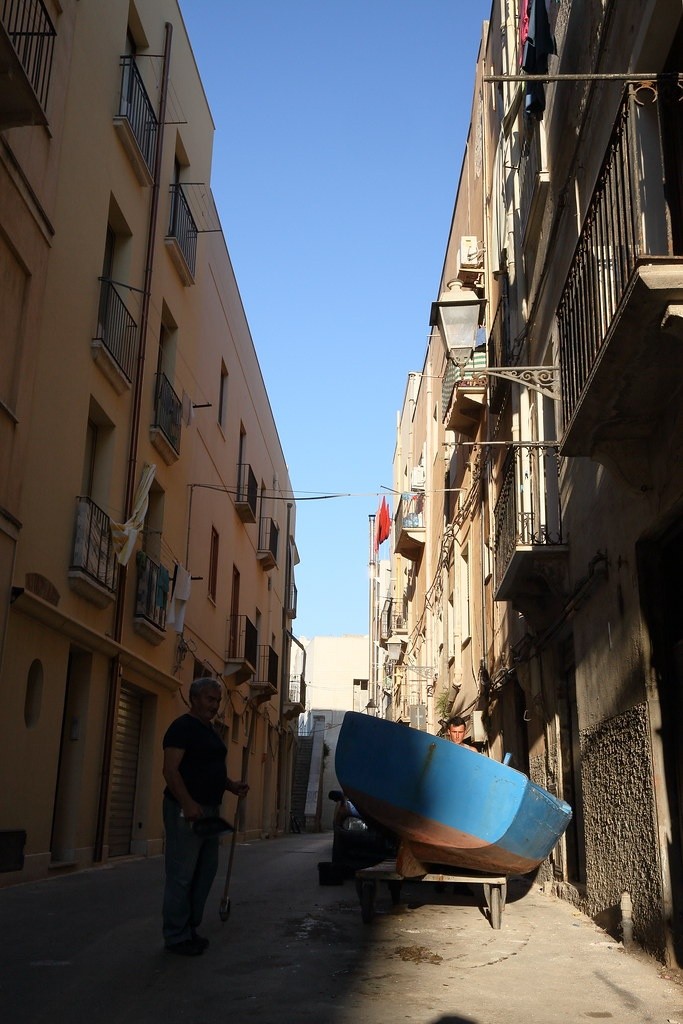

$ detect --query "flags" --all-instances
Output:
[378,496,390,544]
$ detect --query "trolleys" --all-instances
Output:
[356,858,511,930]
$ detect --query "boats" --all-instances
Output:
[335,711,575,878]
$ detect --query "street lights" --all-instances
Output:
[365,698,380,717]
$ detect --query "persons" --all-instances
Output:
[159,678,250,958]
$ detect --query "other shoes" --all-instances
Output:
[165,932,211,958]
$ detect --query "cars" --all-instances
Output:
[329,790,399,875]
